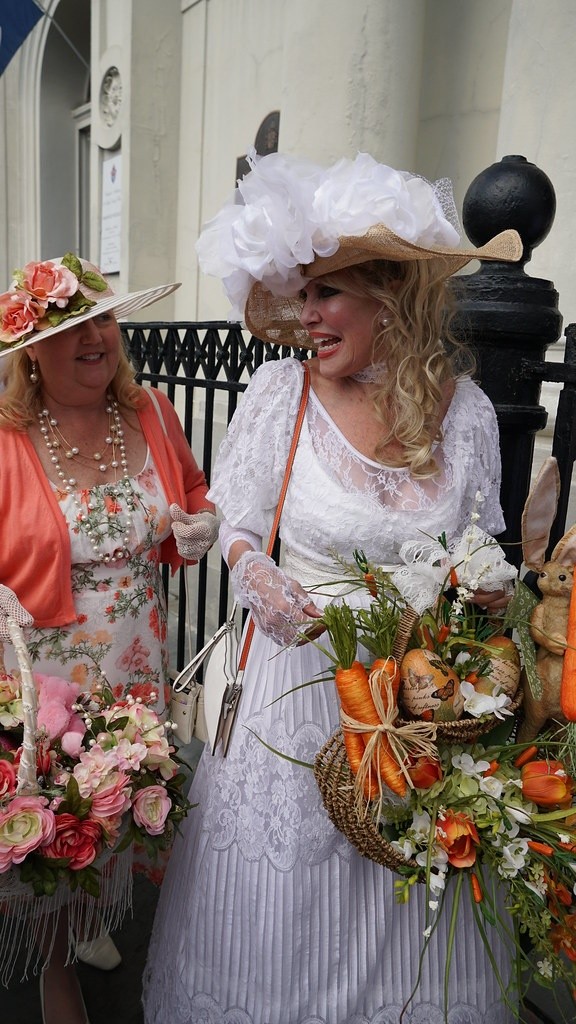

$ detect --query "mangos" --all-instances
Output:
[471,635,520,698]
[401,649,467,720]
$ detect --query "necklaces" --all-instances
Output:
[33,391,133,563]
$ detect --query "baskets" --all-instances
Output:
[314,601,523,879]
[0,614,132,920]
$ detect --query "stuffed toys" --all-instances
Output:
[513,457,575,745]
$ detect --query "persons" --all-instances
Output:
[140,150,528,1024]
[0,250,221,1024]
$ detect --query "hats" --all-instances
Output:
[0,252,182,357]
[193,154,522,350]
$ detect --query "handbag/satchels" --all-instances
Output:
[172,625,244,758]
[167,669,201,745]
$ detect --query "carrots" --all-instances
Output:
[333,657,406,802]
